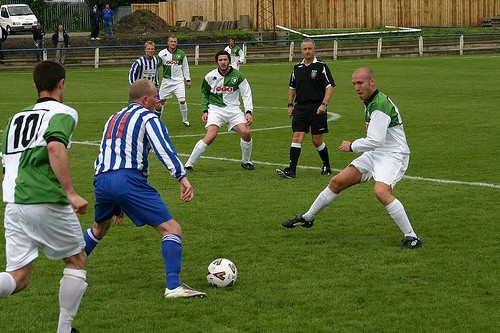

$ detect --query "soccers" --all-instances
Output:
[206,258,237,288]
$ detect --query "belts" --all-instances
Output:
[58,41,64,42]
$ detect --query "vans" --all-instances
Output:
[0,3,41,35]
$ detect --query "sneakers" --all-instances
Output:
[282,214,315,228]
[401,235,422,250]
[164,282,207,300]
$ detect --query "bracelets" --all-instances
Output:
[321,102,327,106]
[288,103,294,108]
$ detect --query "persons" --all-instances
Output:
[281,67,422,248]
[52,24,69,65]
[0,61,89,333]
[225,39,245,70]
[80,77,206,299]
[33,23,45,61]
[157,36,191,128]
[88,4,101,41]
[186,51,254,171]
[274,38,336,177]
[102,6,114,39]
[128,41,162,119]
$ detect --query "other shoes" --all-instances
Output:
[321,165,332,175]
[91,37,95,40]
[276,167,296,178]
[183,119,190,127]
[240,161,256,170]
[96,37,101,40]
[184,166,193,171]
